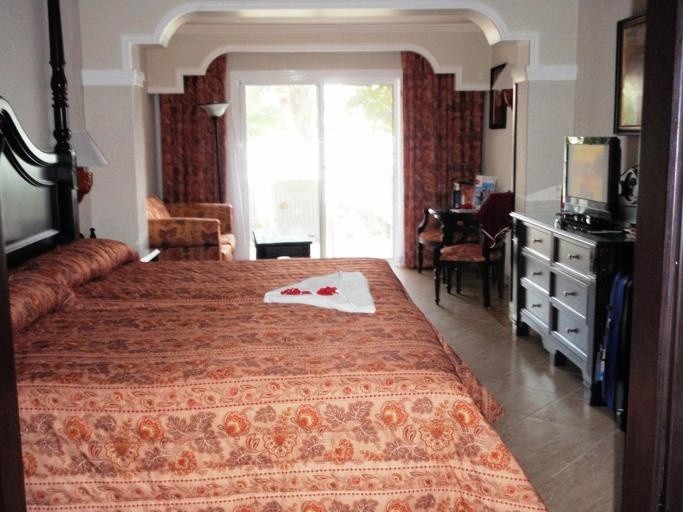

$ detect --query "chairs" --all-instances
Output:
[416,181,514,310]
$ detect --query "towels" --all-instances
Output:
[261,271,377,314]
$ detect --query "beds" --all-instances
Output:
[0,0,549,512]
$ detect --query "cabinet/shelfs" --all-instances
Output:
[509,208,636,406]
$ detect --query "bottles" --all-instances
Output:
[453,182,460,208]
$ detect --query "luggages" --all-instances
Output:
[602,272,634,433]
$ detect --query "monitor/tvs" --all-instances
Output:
[560,136,622,229]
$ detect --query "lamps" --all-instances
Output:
[200,99,230,203]
[75,127,109,203]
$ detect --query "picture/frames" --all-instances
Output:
[489,62,507,130]
[613,13,648,135]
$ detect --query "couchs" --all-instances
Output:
[146,194,236,261]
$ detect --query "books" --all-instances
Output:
[472,174,496,211]
